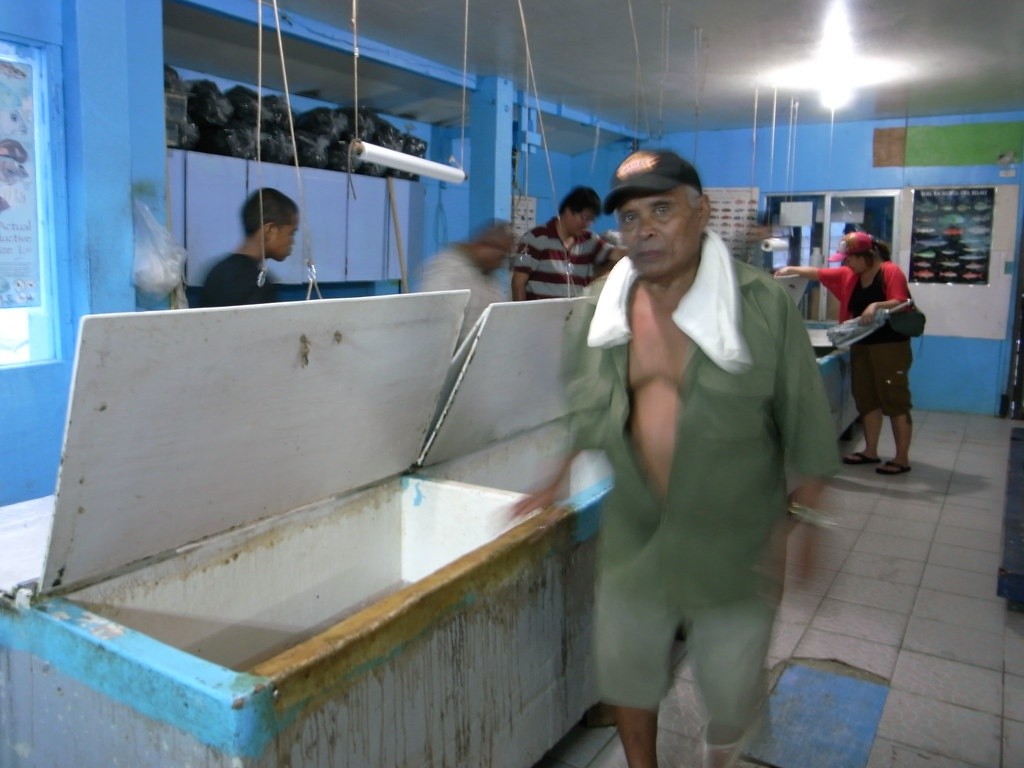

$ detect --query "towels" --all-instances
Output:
[586,224,752,374]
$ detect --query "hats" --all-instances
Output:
[602,150,702,217]
[828,232,873,262]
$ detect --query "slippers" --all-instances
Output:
[843,452,882,464]
[875,460,911,474]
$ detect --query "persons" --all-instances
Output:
[774,231,926,475]
[841,224,858,265]
[412,219,514,350]
[509,148,844,768]
[200,187,299,308]
[510,186,625,300]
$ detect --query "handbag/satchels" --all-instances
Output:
[890,311,927,338]
